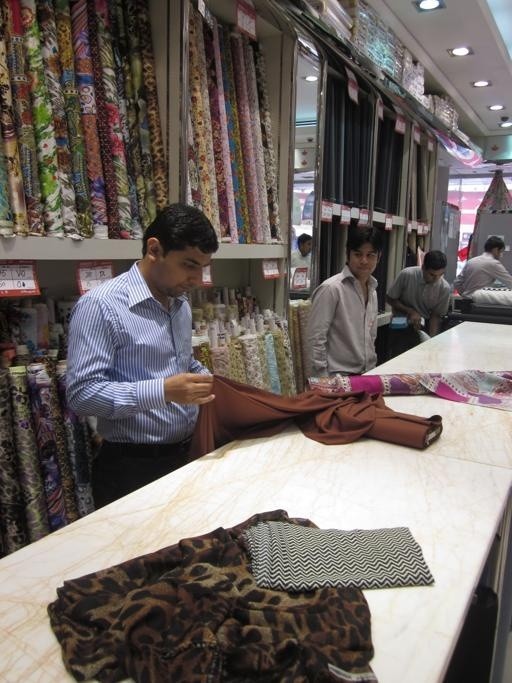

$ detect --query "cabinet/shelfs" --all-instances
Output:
[0,1,291,560]
[321,36,438,364]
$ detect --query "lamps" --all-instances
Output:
[499,116,511,128]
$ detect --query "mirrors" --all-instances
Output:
[289,34,323,298]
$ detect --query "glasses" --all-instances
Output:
[499,116,511,128]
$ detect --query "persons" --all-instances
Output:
[64,201,222,511]
[453,235,512,313]
[291,233,313,269]
[384,250,451,358]
[304,224,384,380]
